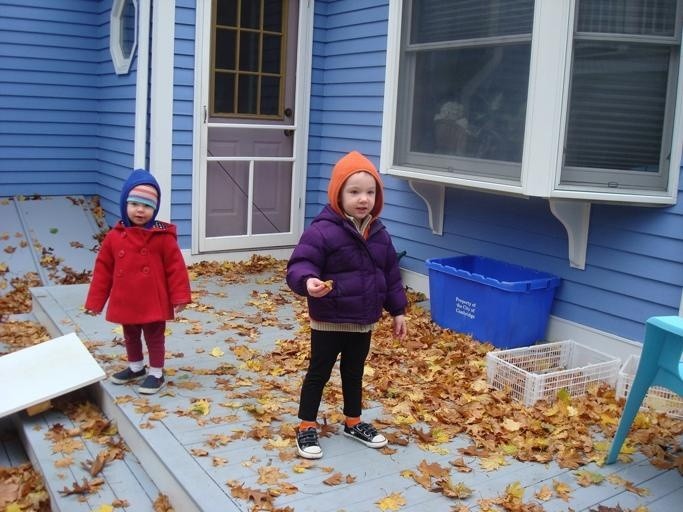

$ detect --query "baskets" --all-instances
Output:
[487,340,621,407]
[615,354,683,419]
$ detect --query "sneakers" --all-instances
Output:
[137,374,165,394]
[343,421,388,448]
[111,366,146,385]
[296,427,322,458]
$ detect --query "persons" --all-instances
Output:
[81,168,192,396]
[284,150,409,460]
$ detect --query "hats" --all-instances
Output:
[127,184,158,210]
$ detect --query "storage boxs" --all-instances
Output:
[425,252,564,353]
